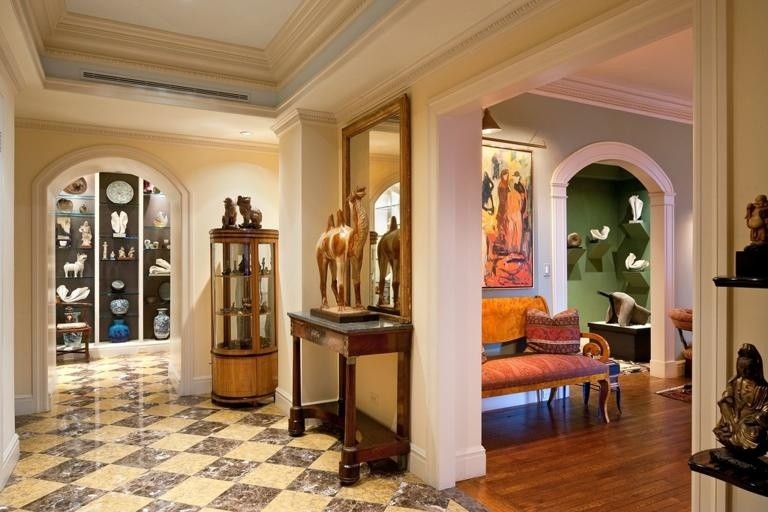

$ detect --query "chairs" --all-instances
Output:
[54,301,93,364]
[665,308,692,390]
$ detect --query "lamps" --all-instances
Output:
[479,109,505,133]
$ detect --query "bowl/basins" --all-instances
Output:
[112,279,125,289]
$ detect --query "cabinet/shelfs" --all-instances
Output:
[99,173,139,343]
[687,277,768,497]
[144,180,171,339]
[208,228,280,408]
[620,222,650,288]
[55,195,96,344]
[566,236,612,263]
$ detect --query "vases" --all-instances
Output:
[153,308,171,340]
[109,297,130,315]
[107,319,132,342]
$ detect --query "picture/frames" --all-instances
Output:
[482,140,536,290]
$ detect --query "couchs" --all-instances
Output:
[482,296,610,425]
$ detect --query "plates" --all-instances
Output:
[63,177,88,195]
[158,281,170,302]
[105,180,136,204]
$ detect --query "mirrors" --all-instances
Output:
[339,95,412,324]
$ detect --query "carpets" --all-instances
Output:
[656,383,692,404]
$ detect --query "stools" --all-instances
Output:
[582,356,624,418]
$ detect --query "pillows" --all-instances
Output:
[524,306,581,355]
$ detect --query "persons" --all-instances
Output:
[496,169,527,256]
[713,345,767,459]
[745,193,767,244]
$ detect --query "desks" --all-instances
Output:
[288,312,409,484]
[586,319,650,362]
